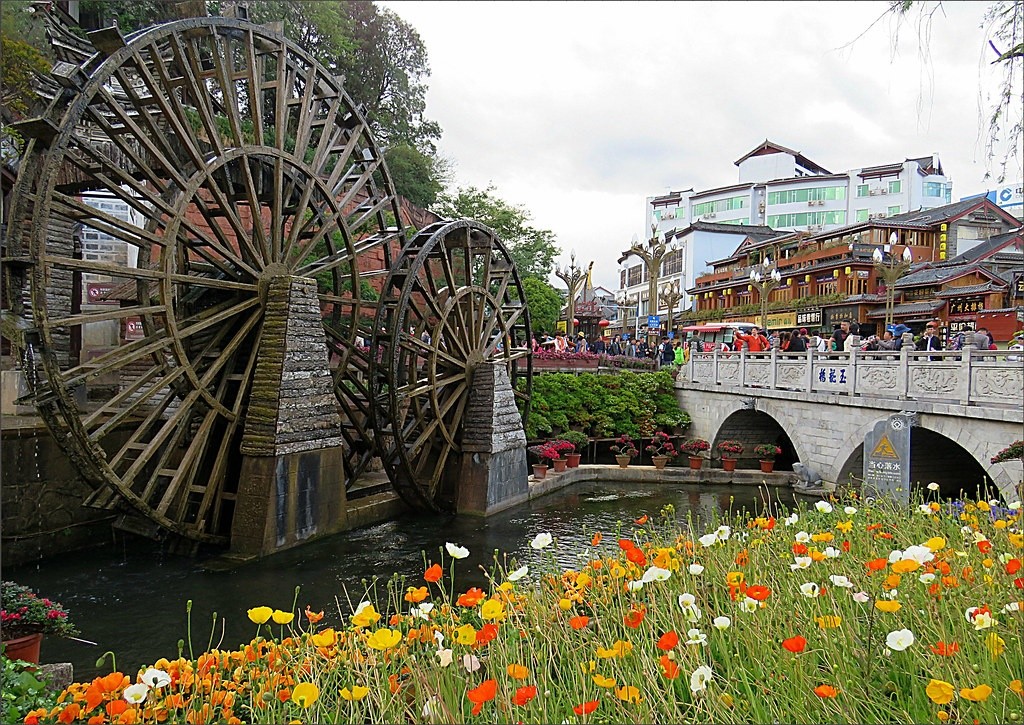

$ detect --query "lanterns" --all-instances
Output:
[748,285,752,292]
[941,224,947,231]
[573,318,579,327]
[709,292,713,298]
[939,252,946,259]
[598,319,610,328]
[690,296,693,301]
[722,289,726,296]
[940,243,946,251]
[833,270,838,278]
[695,295,698,300]
[727,289,731,294]
[845,267,851,274]
[786,278,791,286]
[704,293,708,299]
[805,275,810,283]
[939,233,946,242]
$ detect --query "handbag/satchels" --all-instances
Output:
[760,342,765,350]
[664,351,675,361]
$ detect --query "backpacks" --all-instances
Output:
[949,333,963,349]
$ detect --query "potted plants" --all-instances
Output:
[555,431,590,467]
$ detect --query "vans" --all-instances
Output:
[705,322,762,333]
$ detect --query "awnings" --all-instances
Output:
[682,325,726,332]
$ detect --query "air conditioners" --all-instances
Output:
[808,225,823,229]
[807,200,824,205]
[760,202,765,213]
[868,213,886,218]
[661,214,674,220]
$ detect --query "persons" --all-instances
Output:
[341,324,432,346]
[1009,334,1024,350]
[730,319,997,362]
[687,330,705,352]
[516,330,691,374]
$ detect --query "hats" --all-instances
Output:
[800,328,807,334]
[894,324,911,336]
[1018,334,1024,339]
[578,332,584,337]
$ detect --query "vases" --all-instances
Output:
[532,465,549,479]
[721,459,737,471]
[652,457,668,469]
[553,459,568,472]
[759,460,775,472]
[615,455,630,468]
[688,456,704,469]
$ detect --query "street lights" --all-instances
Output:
[657,276,683,343]
[614,290,638,349]
[873,232,912,333]
[555,248,594,342]
[631,214,678,348]
[750,257,781,330]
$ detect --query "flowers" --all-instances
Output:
[715,439,745,457]
[753,444,781,459]
[528,445,559,464]
[609,434,639,456]
[646,431,676,455]
[681,439,711,455]
[543,440,575,459]
